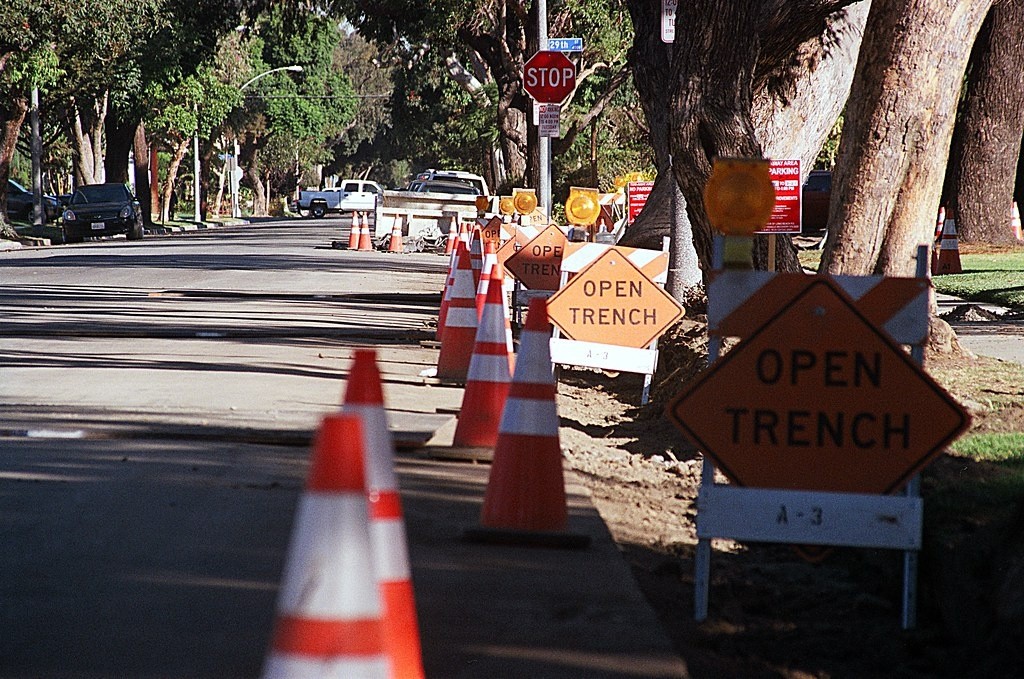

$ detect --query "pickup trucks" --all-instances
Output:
[293,179,385,219]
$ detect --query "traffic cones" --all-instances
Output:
[255,412,403,679]
[418,263,510,465]
[451,297,597,555]
[344,210,358,250]
[330,347,429,679]
[421,220,518,387]
[935,206,948,243]
[357,212,377,252]
[436,215,458,256]
[383,212,409,254]
[1012,201,1022,239]
[934,208,964,276]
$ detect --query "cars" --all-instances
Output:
[61,183,144,243]
[6,179,61,225]
[393,169,489,196]
[800,171,835,230]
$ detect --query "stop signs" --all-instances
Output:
[522,50,577,106]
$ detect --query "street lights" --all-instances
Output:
[228,66,303,216]
